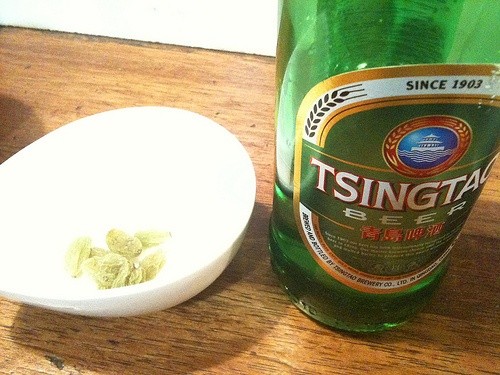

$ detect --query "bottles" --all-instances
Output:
[267,0,500,331]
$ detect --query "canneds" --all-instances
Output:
[271,0,500,333]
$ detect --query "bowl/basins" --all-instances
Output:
[0,106,256,317]
